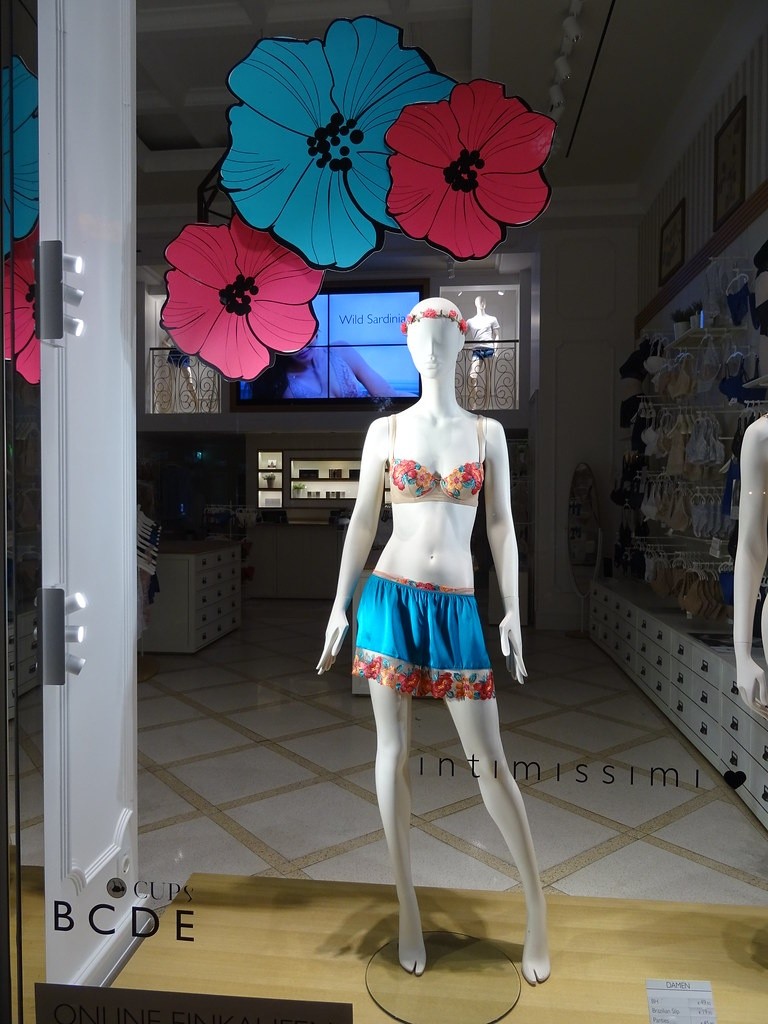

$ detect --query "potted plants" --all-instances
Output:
[263,474,276,489]
[293,482,306,498]
[686,301,702,328]
[670,308,688,340]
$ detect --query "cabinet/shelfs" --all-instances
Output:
[137,540,243,653]
[14,595,41,698]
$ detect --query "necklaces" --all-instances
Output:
[287,356,323,396]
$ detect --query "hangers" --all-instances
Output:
[613,396,767,602]
[637,254,759,368]
[381,503,392,519]
[234,505,260,515]
[205,504,233,514]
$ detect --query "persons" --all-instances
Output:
[241,297,419,399]
[314,294,557,988]
[733,411,768,717]
[463,294,501,412]
[161,334,202,414]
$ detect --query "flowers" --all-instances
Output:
[400,307,468,334]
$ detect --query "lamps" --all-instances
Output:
[64,652,85,675]
[64,593,86,615]
[65,626,84,643]
